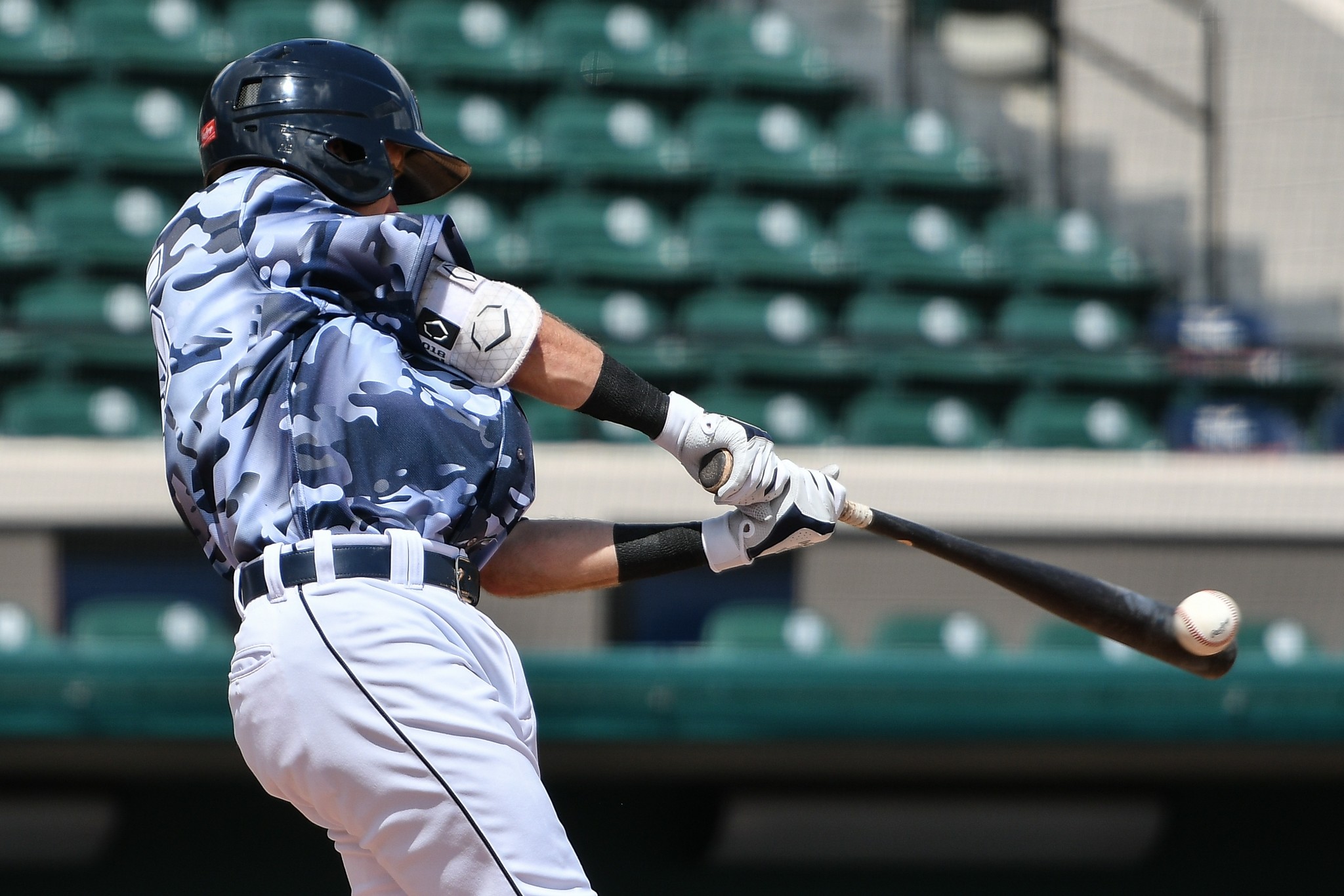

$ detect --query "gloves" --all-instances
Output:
[651,390,791,506]
[702,464,847,572]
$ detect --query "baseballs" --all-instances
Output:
[1174,589,1240,657]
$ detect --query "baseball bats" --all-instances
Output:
[698,443,1240,680]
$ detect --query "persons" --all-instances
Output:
[145,39,847,896]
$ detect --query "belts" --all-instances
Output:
[237,545,480,606]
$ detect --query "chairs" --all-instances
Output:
[0,0,1344,462]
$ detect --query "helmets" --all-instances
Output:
[198,36,473,208]
